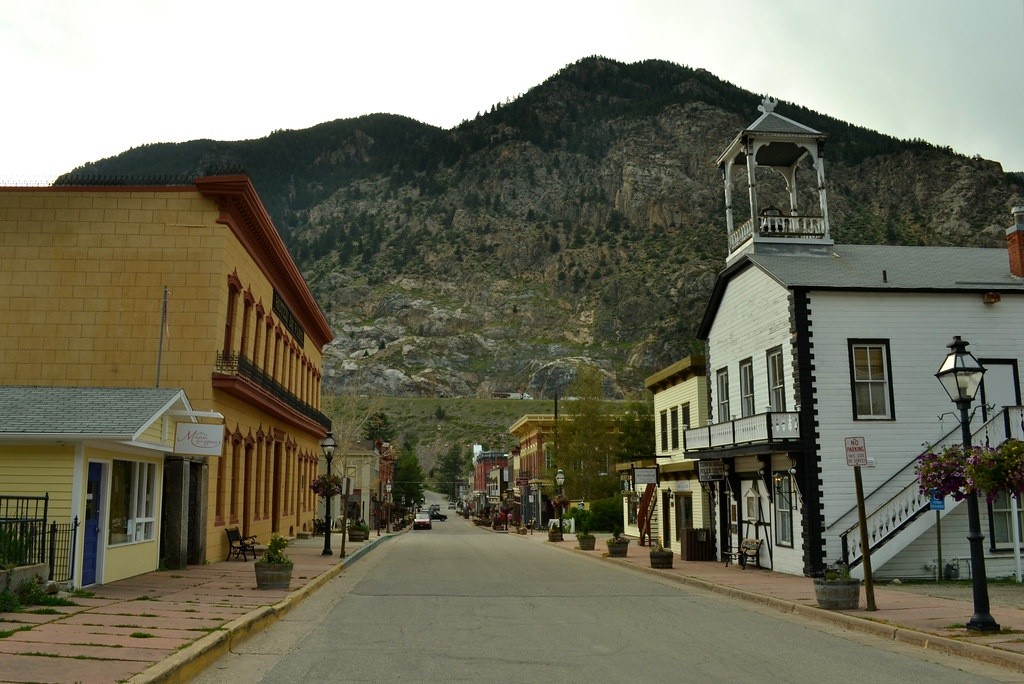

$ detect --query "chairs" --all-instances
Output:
[813,564,860,610]
[312,518,325,537]
[724,538,763,570]
[224,526,256,562]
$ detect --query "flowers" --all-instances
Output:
[650,536,669,552]
[607,523,630,543]
[914,438,1024,503]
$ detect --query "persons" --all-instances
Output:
[349,499,362,521]
[499,512,506,525]
[507,511,513,526]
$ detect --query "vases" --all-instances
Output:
[650,551,673,569]
[607,540,628,557]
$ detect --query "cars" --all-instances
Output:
[421,510,447,521]
[413,513,432,529]
[448,503,456,509]
[430,504,440,511]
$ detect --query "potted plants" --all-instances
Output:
[348,519,369,541]
[548,522,561,542]
[576,527,596,550]
[255,535,293,590]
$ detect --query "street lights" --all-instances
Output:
[933,335,1001,630]
[385,479,391,533]
[320,432,338,556]
[502,491,508,530]
[401,496,405,526]
[555,470,565,540]
[411,498,414,514]
